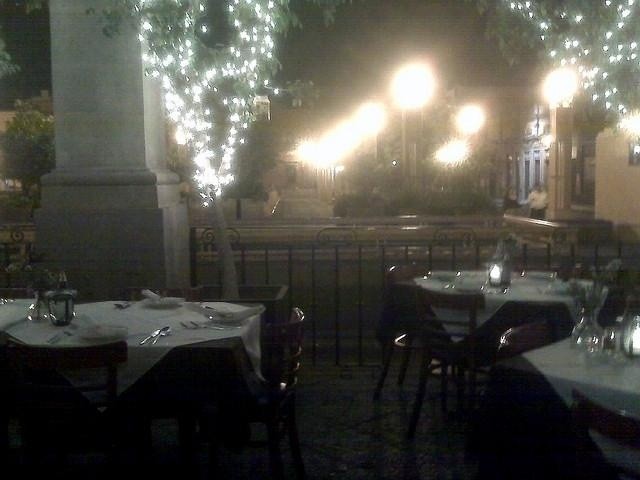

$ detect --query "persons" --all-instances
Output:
[492,186,524,260]
[518,180,551,219]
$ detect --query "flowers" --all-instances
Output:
[4,262,70,295]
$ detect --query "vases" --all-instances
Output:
[26,293,51,324]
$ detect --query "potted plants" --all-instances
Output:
[83,0,303,323]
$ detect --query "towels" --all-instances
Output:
[140,288,253,319]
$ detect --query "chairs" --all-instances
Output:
[0,337,134,480]
[193,306,310,479]
[367,254,640,480]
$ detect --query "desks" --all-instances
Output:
[1,293,267,480]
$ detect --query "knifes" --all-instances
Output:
[139,328,160,345]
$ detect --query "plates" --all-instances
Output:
[204,305,266,324]
[78,325,129,341]
[142,296,186,309]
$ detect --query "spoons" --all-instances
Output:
[151,326,171,345]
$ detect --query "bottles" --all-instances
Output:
[25,290,49,322]
[568,305,604,353]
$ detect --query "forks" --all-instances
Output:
[177,319,241,331]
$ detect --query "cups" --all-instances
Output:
[605,323,630,366]
[542,269,558,283]
[581,331,601,364]
